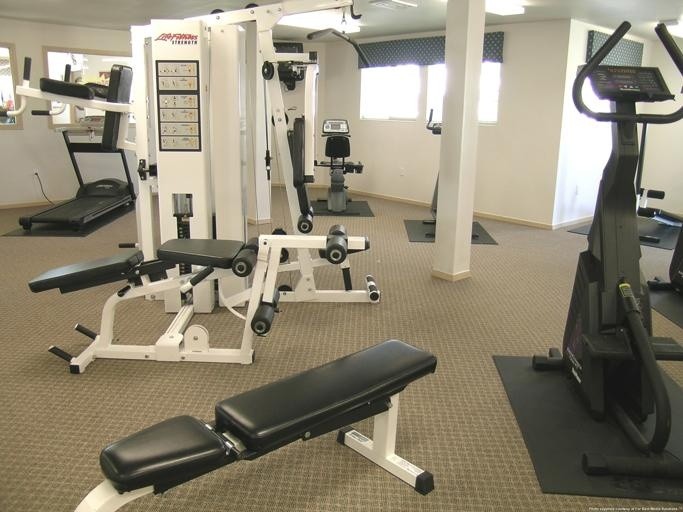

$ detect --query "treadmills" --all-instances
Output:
[18,116,137,237]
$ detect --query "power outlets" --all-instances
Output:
[34,170,39,177]
